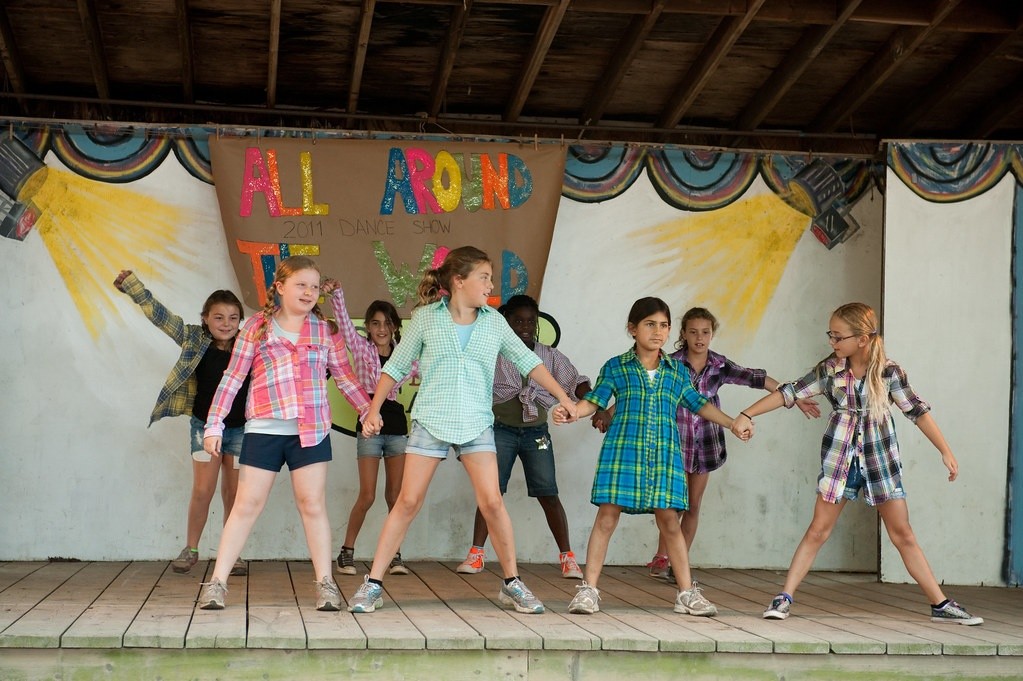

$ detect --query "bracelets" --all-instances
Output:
[740,412,751,420]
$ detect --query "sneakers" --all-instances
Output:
[172,545,200,575]
[229,555,250,576]
[559,550,584,579]
[313,576,341,611]
[389,553,409,574]
[498,574,546,613]
[197,576,229,609]
[667,565,677,583]
[762,591,794,619]
[568,579,602,613]
[348,573,384,613]
[673,581,719,616]
[930,598,984,625]
[336,545,357,575]
[456,547,487,573]
[647,553,670,579]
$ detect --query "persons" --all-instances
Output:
[112,268,254,576]
[347,244,580,614]
[322,274,409,576]
[456,298,609,579]
[729,302,984,624]
[590,306,821,584]
[552,295,748,617]
[200,254,384,611]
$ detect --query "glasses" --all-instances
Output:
[826,330,861,343]
